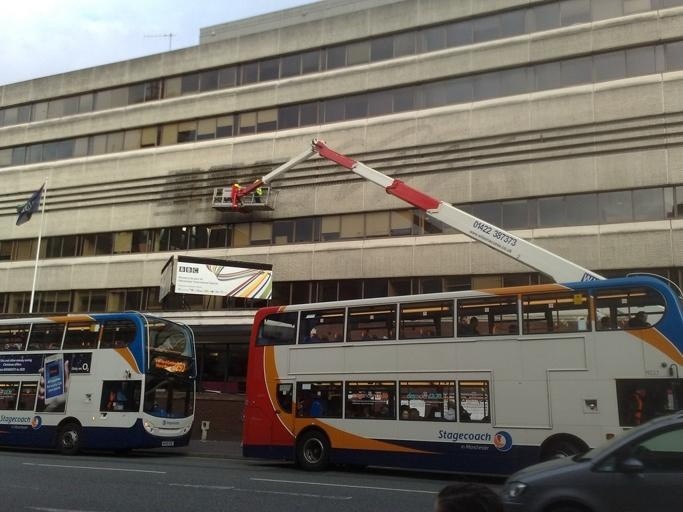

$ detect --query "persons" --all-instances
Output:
[283,387,420,420]
[626,310,653,327]
[459,316,473,336]
[232,179,244,205]
[254,177,263,204]
[117,390,130,401]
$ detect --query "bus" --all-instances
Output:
[0,310,197,456]
[243,275,683,470]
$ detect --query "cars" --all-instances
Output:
[501,410,683,512]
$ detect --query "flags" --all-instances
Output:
[16,181,45,226]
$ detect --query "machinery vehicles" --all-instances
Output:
[210,139,609,283]
